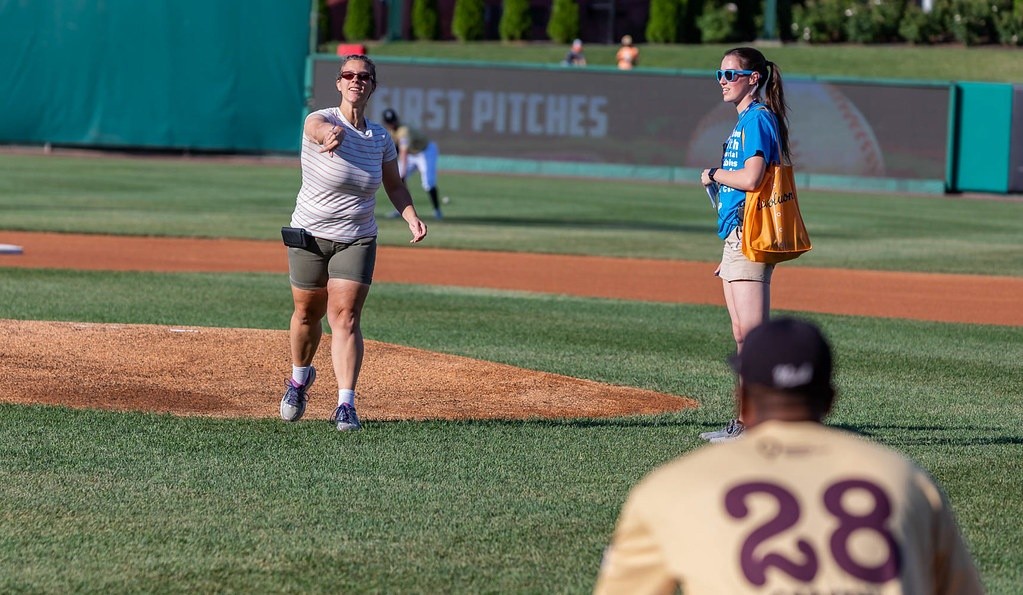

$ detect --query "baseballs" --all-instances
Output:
[442,197,450,205]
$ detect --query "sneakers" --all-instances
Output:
[329,403,360,430]
[280,365,316,422]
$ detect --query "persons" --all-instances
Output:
[565,39,586,66]
[380,108,444,218]
[698,45,788,442]
[593,317,986,595]
[281,54,426,430]
[615,35,639,69]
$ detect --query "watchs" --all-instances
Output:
[708,167,718,182]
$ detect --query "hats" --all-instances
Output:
[728,316,833,390]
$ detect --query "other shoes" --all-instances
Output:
[700,419,745,444]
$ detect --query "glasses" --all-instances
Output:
[338,71,371,81]
[716,70,754,82]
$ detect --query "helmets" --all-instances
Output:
[384,109,396,122]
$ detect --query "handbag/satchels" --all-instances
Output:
[741,106,812,262]
